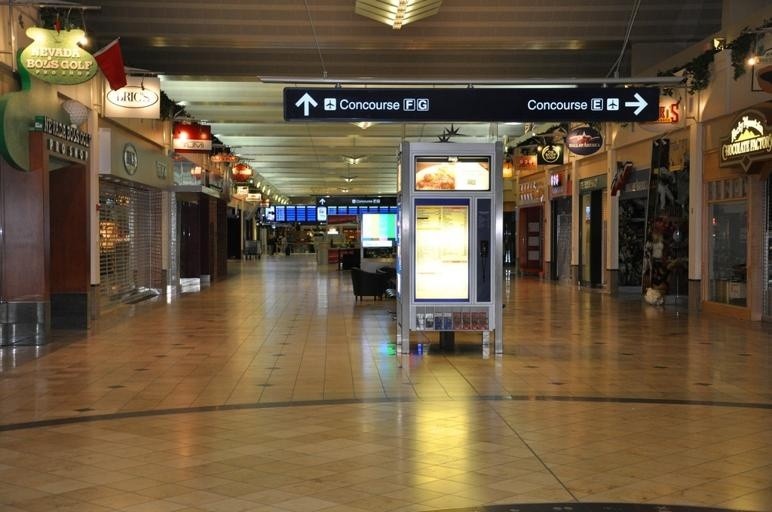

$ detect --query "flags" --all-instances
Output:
[93,40,128,91]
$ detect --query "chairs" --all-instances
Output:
[376,268,395,298]
[350,267,387,300]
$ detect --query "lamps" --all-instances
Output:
[337,137,368,193]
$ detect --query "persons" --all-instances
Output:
[644,281,665,306]
[267,233,324,256]
